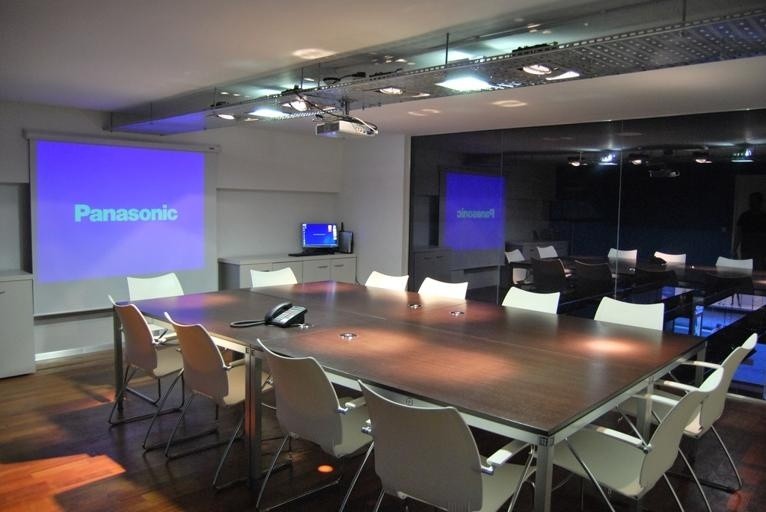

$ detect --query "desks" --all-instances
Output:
[115,281,710,512]
[0,269,37,380]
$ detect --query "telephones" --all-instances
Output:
[265,301,307,327]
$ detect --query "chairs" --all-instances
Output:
[364,270,410,292]
[108,294,229,452]
[611,333,757,512]
[529,367,724,512]
[501,245,754,305]
[501,286,561,313]
[356,377,537,512]
[163,310,294,490]
[249,266,299,288]
[254,338,375,511]
[124,273,185,404]
[417,277,469,300]
[594,296,665,332]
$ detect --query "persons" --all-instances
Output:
[732,192,765,273]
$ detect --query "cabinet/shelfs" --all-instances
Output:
[303,257,356,283]
[241,261,302,288]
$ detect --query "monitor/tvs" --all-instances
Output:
[302,223,339,254]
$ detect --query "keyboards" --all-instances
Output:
[289,252,328,257]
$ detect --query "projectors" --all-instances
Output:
[314,117,379,138]
